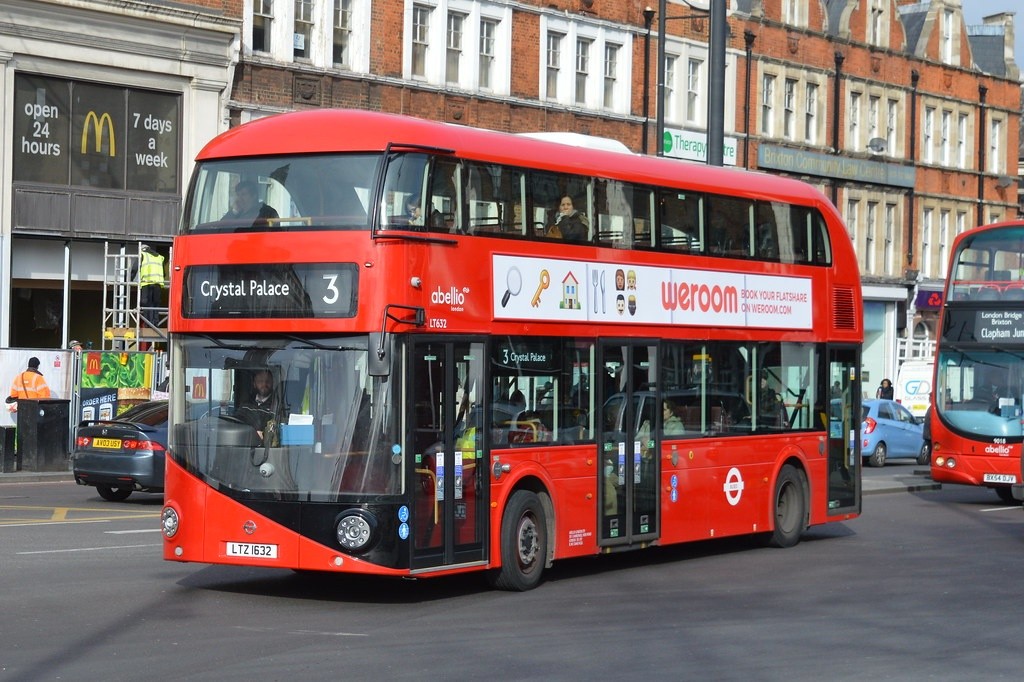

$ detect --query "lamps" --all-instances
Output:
[994,175,1012,189]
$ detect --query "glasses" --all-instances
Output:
[408,207,417,214]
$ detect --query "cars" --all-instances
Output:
[861,398,931,466]
[450,398,750,447]
[72,399,274,500]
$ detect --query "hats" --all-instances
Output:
[67,340,82,349]
[141,244,151,249]
[28,357,40,367]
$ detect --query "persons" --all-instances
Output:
[219,370,277,440]
[220,181,280,228]
[11,357,50,399]
[634,399,685,452]
[543,192,589,242]
[404,192,448,227]
[876,378,894,400]
[130,244,167,327]
[830,380,842,399]
[761,372,789,427]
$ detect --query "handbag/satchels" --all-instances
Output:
[547,227,563,240]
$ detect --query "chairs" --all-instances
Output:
[488,401,792,440]
[975,284,1024,300]
[387,212,757,260]
[277,380,305,416]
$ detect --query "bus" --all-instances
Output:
[931,220,1024,506]
[159,107,863,593]
